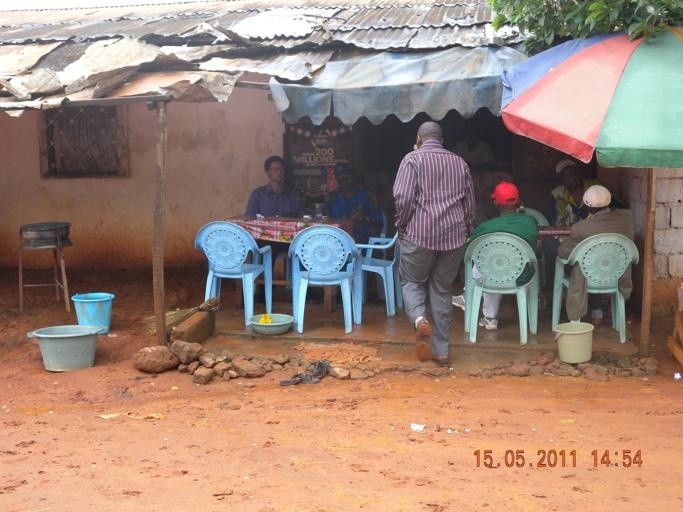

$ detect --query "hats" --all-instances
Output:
[489,181,520,207]
[555,158,576,175]
[582,184,612,208]
[334,163,352,181]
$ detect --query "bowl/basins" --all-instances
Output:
[16,221,78,244]
[247,313,293,335]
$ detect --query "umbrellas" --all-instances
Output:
[498,17,683,357]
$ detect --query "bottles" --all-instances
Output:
[518,202,525,214]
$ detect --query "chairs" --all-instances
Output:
[550,232,639,344]
[194,221,273,326]
[288,225,363,334]
[378,209,389,299]
[463,232,540,346]
[355,230,403,317]
[274,250,295,290]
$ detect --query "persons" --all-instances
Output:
[324,161,382,303]
[243,156,304,304]
[542,184,636,326]
[548,159,625,228]
[392,122,477,367]
[451,181,540,331]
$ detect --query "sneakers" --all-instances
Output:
[478,316,498,330]
[431,353,451,367]
[415,320,432,362]
[452,295,466,311]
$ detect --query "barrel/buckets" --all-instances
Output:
[71,292,116,335]
[552,318,595,364]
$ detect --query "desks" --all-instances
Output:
[225,215,367,313]
[469,223,572,252]
